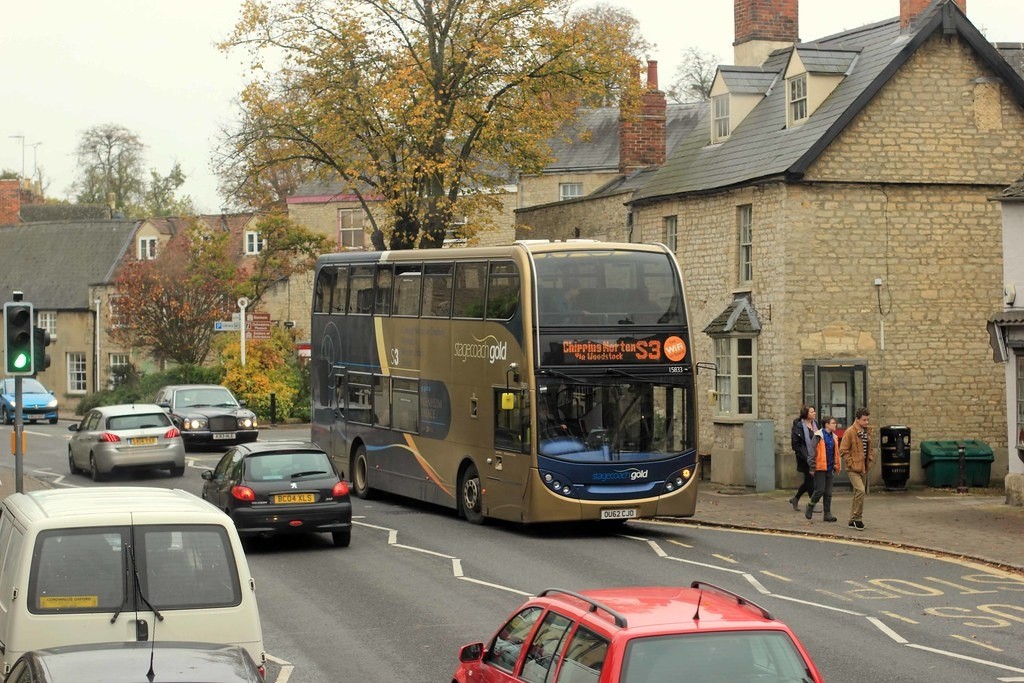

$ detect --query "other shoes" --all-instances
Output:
[825,513,837,521]
[810,507,822,513]
[806,503,813,519]
[849,520,867,531]
[790,497,800,510]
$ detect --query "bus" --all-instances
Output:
[309,237,719,538]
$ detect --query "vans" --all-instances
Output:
[0,486,268,683]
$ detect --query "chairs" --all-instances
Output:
[144,533,173,549]
[353,285,662,321]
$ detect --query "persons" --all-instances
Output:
[804,415,841,522]
[547,287,590,324]
[839,406,874,530]
[537,401,568,438]
[788,404,822,512]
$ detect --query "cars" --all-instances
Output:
[151,385,258,453]
[200,440,354,551]
[449,579,825,683]
[0,378,58,425]
[68,404,186,483]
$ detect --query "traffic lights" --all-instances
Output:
[4,302,34,376]
[33,325,52,373]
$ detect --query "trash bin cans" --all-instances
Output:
[879,424,912,491]
[918,440,995,487]
